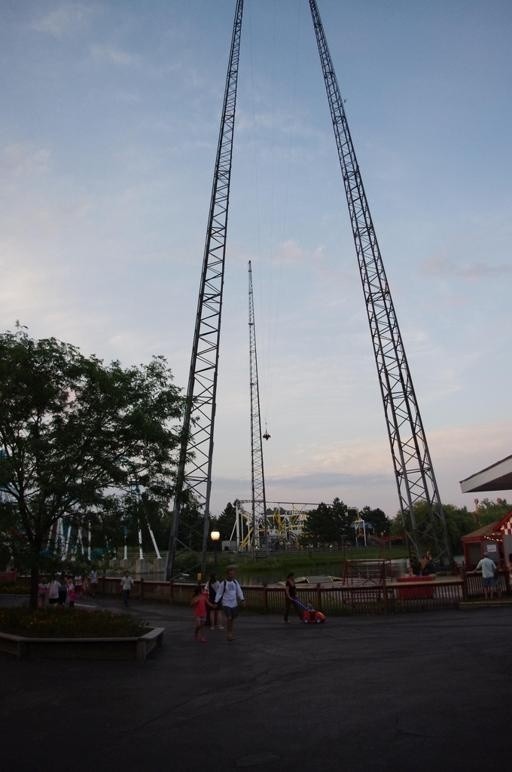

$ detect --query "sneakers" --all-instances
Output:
[228,634,236,641]
[193,634,208,643]
[211,625,224,631]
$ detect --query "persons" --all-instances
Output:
[214,568,246,641]
[119,571,134,606]
[189,589,216,644]
[35,564,99,608]
[204,574,225,630]
[406,548,435,577]
[473,551,497,601]
[282,572,304,624]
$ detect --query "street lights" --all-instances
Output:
[209,529,220,567]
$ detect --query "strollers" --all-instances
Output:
[292,596,326,623]
